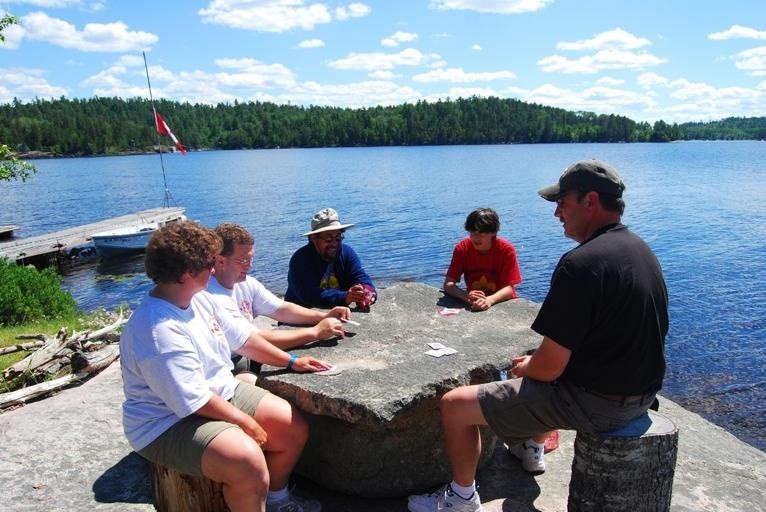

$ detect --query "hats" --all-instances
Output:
[301,206,356,238]
[536,155,627,204]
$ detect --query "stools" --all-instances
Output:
[146,458,231,512]
[562,406,678,512]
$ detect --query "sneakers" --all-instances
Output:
[265,492,322,512]
[407,482,482,512]
[506,435,548,474]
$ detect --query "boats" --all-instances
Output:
[94,254,147,277]
[89,210,204,259]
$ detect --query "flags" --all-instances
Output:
[155,111,187,156]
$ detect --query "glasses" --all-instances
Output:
[224,255,254,266]
[194,259,217,270]
[316,233,346,243]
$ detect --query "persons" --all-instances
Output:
[444,207,522,312]
[278,208,377,330]
[406,160,670,512]
[118,220,323,511]
[205,221,352,386]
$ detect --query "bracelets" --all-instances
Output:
[286,352,300,370]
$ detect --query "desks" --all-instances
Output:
[254,277,545,511]
